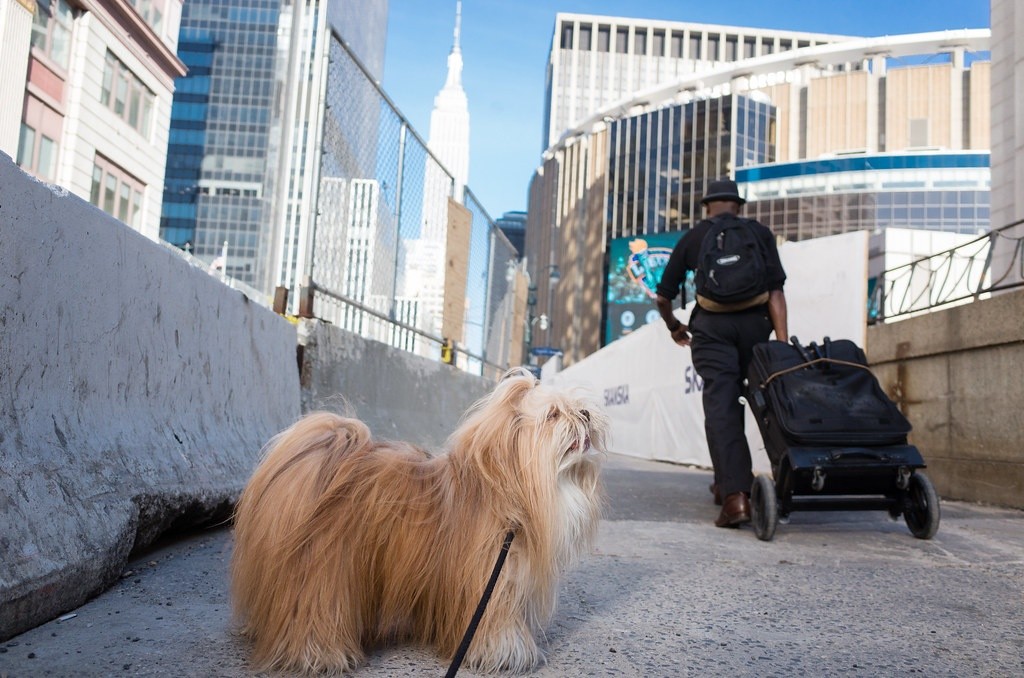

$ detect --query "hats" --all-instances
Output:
[700,181,746,206]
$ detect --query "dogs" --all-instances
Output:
[212,367,613,678]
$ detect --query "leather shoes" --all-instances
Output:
[714,493,751,526]
[709,483,727,506]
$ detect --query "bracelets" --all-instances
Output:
[667,320,680,332]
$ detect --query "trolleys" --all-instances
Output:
[740,336,942,542]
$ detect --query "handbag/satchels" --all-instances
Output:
[746,335,914,465]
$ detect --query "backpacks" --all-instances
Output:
[694,214,766,305]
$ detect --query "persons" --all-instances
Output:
[656,182,788,527]
